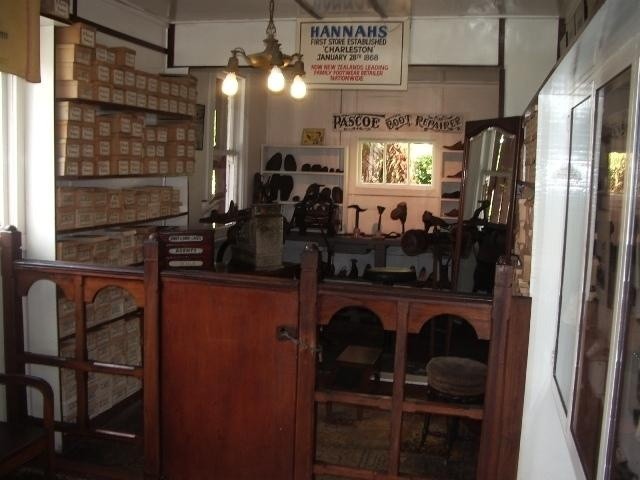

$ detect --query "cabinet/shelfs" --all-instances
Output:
[440,149,459,223]
[26,21,193,421]
[261,142,349,231]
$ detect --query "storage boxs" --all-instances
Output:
[54,22,201,270]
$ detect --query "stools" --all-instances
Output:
[419,356,487,465]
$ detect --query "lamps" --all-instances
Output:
[220,0,308,100]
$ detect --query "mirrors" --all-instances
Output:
[450,117,524,294]
